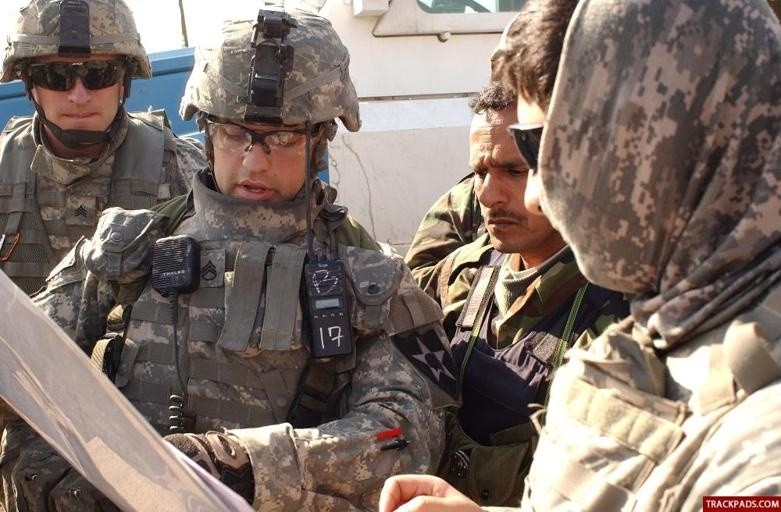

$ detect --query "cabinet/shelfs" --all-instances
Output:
[0,46,330,188]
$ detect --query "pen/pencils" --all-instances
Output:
[381,439,412,451]
[377,427,402,441]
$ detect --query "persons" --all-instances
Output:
[403,171,488,269]
[0,1,208,296]
[0,0,464,512]
[424,85,632,508]
[377,1,780,512]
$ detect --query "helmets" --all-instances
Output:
[179,8,361,132]
[0,0,151,83]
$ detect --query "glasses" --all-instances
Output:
[508,124,543,171]
[203,120,315,157]
[27,60,127,91]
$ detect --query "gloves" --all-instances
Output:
[164,431,254,504]
